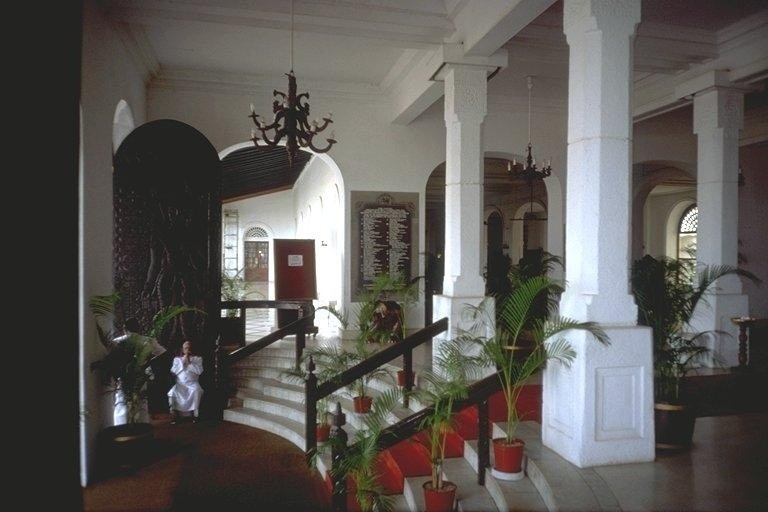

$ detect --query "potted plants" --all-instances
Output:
[480,250,611,473]
[411,335,462,511]
[289,353,332,442]
[628,253,763,451]
[216,262,265,348]
[332,332,372,415]
[88,294,208,468]
[367,267,416,387]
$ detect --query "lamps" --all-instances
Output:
[248,0,340,169]
[504,75,551,183]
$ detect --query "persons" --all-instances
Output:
[95,317,167,426]
[167,341,205,425]
